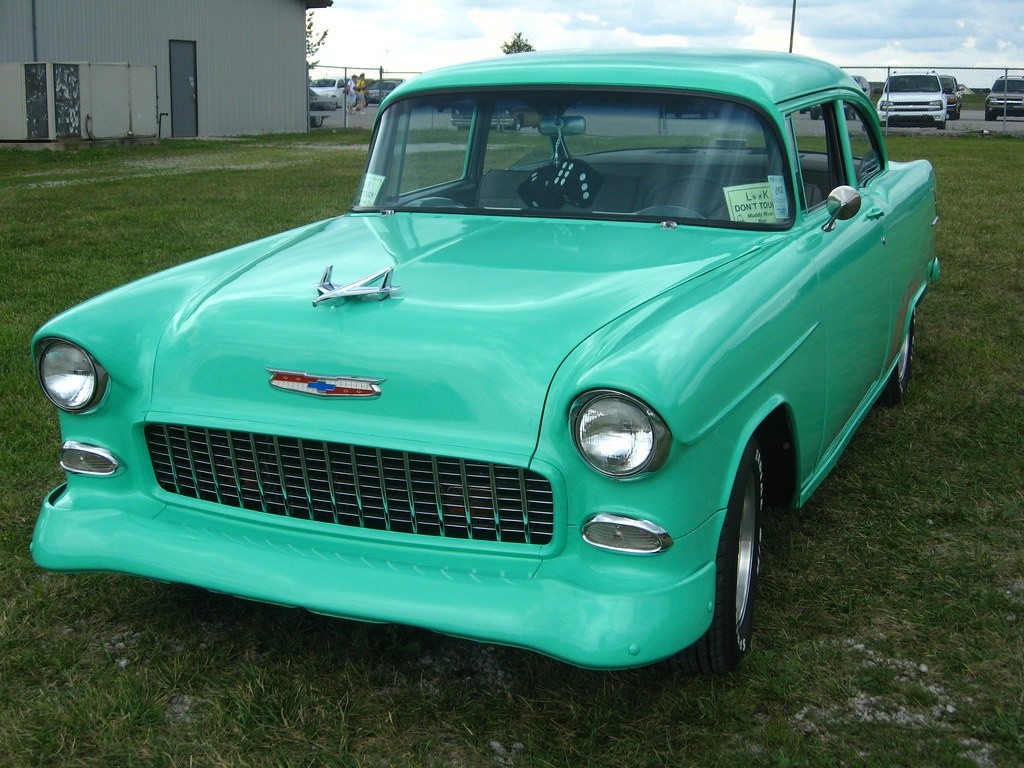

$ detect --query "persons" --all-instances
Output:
[345,73,366,114]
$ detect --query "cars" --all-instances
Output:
[449,100,566,133]
[306,87,334,128]
[809,75,871,120]
[362,80,401,105]
[307,78,357,111]
[658,93,726,120]
[26,43,940,678]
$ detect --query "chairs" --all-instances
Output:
[475,169,824,223]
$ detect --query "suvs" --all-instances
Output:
[873,69,950,130]
[916,75,964,119]
[983,74,1024,120]
